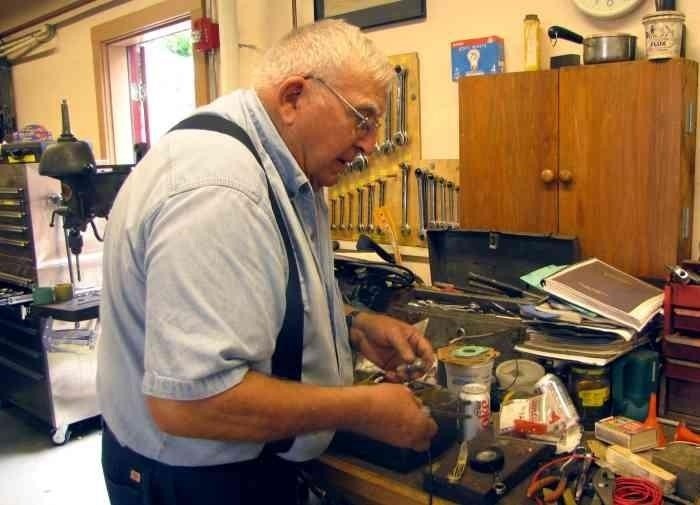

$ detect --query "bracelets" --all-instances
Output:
[345,311,361,349]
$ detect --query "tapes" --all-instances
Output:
[469,447,504,473]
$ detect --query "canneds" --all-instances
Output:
[458,384,491,441]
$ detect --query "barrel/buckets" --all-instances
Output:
[641,10,685,60]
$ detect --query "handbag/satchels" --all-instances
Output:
[147,460,345,504]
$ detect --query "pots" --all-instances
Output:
[545,24,635,62]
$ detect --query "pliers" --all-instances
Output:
[527,462,580,503]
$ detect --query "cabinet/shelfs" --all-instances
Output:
[455,58,700,285]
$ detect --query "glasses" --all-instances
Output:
[303,74,381,132]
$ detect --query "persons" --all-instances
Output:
[96,18,439,505]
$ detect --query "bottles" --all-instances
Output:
[524,14,542,72]
[571,366,609,424]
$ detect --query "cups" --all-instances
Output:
[640,10,686,63]
[443,362,494,396]
[31,284,72,306]
